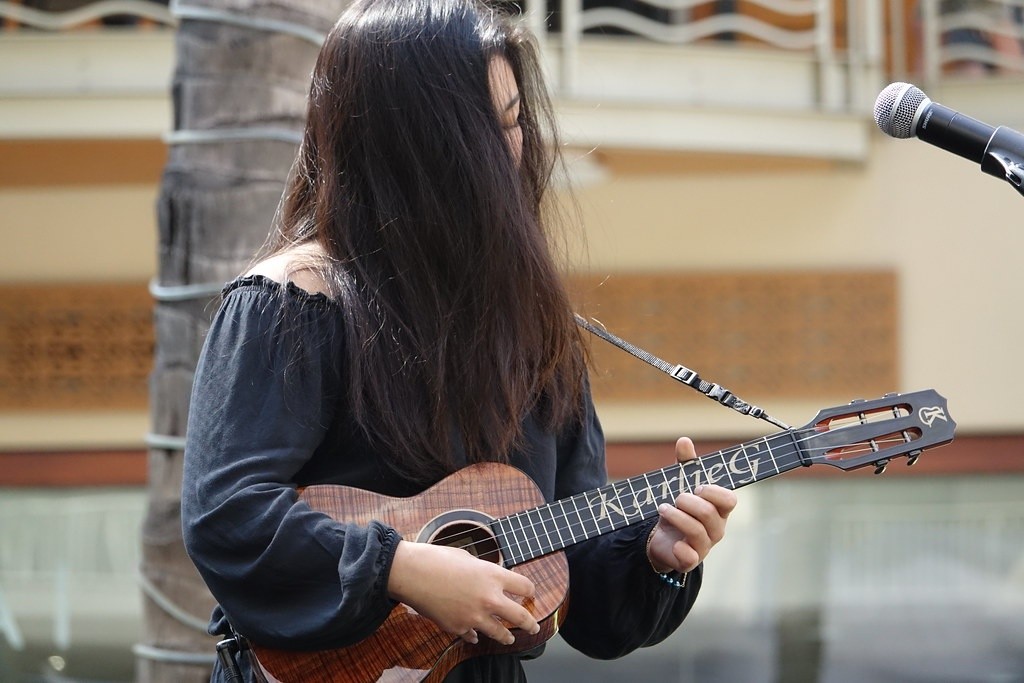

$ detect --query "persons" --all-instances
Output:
[180,1,736,683]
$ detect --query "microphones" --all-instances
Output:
[873,82,1024,198]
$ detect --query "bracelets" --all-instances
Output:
[647,522,688,588]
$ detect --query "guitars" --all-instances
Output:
[250,390,955,683]
